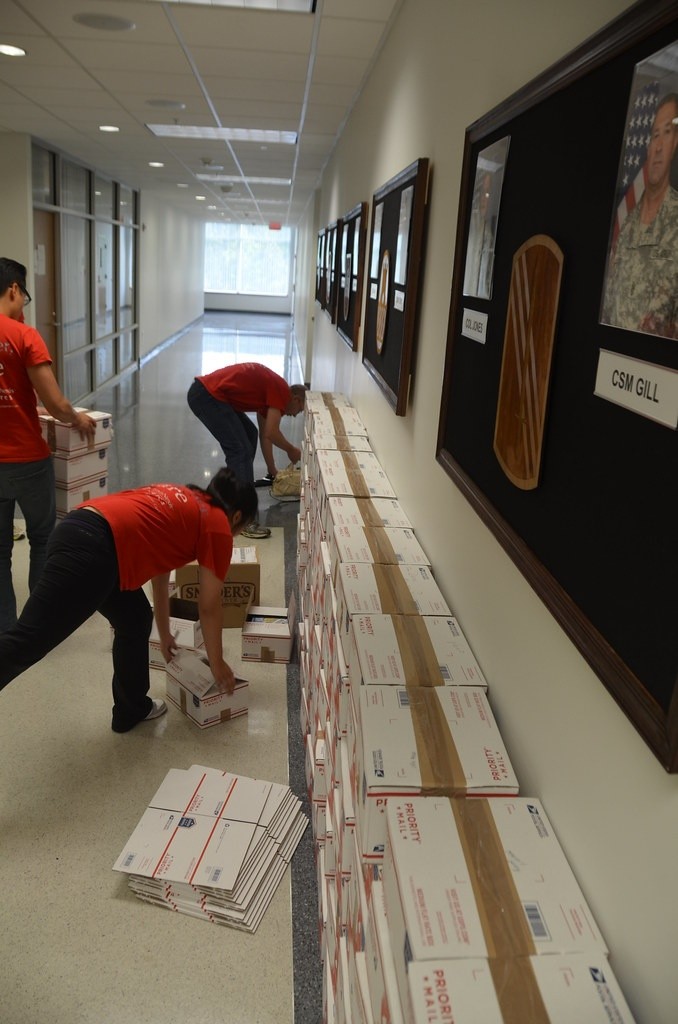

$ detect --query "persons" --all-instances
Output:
[187,361,308,537]
[0,257,98,634]
[0,464,258,733]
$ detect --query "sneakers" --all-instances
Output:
[240,516,270,538]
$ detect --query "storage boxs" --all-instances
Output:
[296,390,635,1023]
[37,406,113,457]
[241,586,296,664]
[50,448,109,492]
[54,478,108,520]
[175,544,260,628]
[165,647,249,729]
[110,567,207,671]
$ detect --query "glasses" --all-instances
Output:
[10,280,31,306]
[298,398,303,413]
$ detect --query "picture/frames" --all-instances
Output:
[317,186,413,285]
[597,40,678,341]
[462,135,510,300]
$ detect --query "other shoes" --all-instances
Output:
[111,696,167,733]
